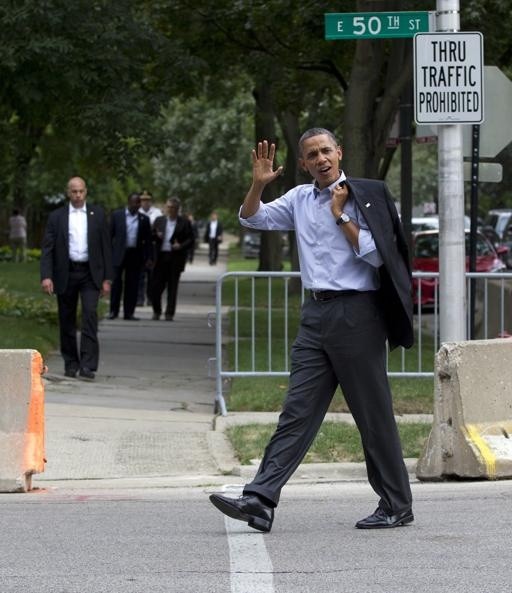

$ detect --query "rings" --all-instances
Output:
[336,186,343,190]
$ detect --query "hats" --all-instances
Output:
[66,369,76,377]
[80,367,94,378]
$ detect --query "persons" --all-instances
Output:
[108,189,198,320]
[203,212,222,264]
[210,126,416,533]
[41,175,111,379]
[9,206,29,263]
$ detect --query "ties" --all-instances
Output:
[310,291,355,300]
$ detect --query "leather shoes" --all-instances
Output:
[109,312,172,321]
[356,507,414,529]
[210,494,274,531]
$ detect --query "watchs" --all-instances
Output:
[335,213,350,227]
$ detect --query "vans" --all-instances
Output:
[482,207,510,237]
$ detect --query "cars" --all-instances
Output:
[242,229,288,257]
[408,230,508,307]
[412,216,440,230]
[463,214,480,235]
